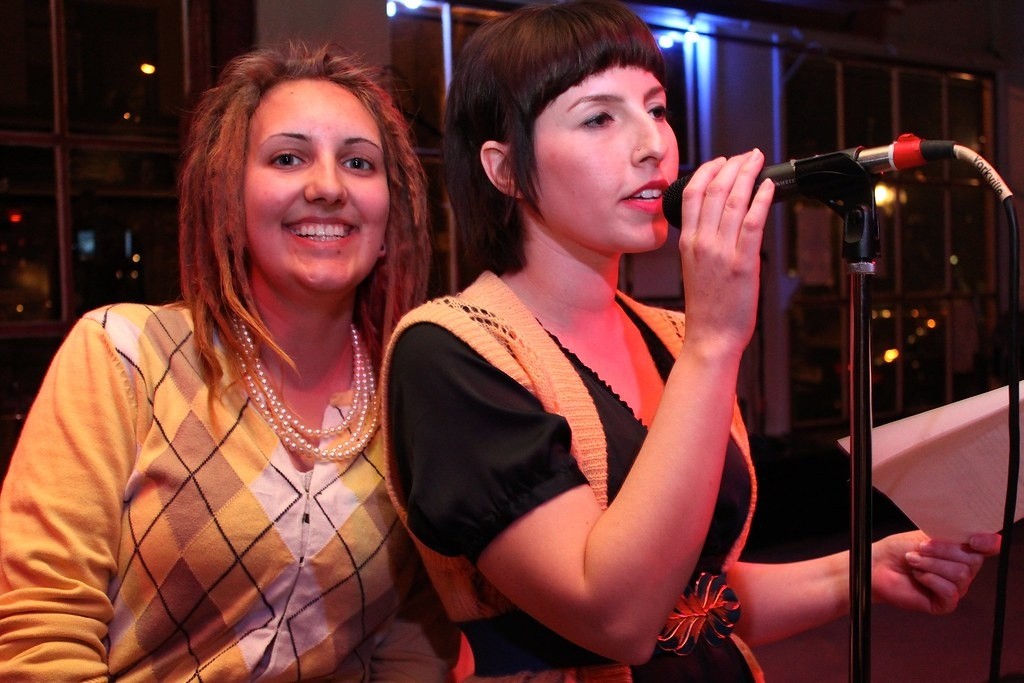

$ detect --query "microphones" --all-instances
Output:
[662,134,1013,230]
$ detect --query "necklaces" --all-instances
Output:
[227,306,383,465]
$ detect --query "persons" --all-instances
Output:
[380,1,1006,682]
[1,45,465,683]
